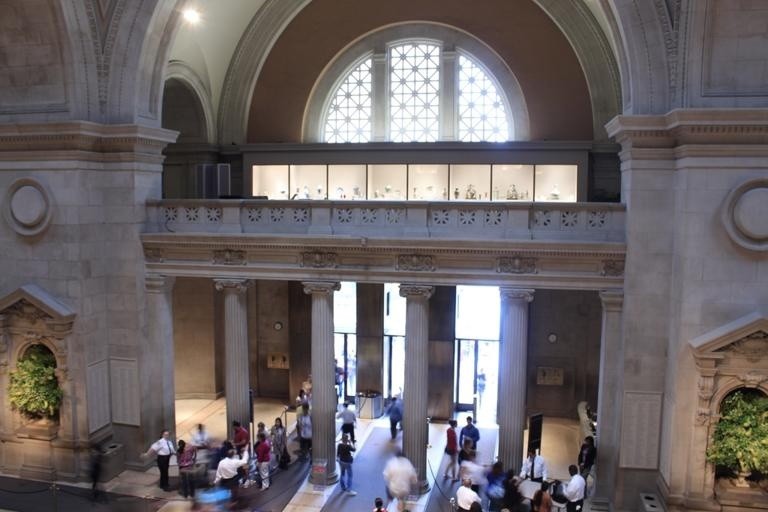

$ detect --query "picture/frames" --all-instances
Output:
[535,365,565,386]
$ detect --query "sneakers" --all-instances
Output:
[443,472,459,482]
[340,487,358,496]
[259,485,270,492]
